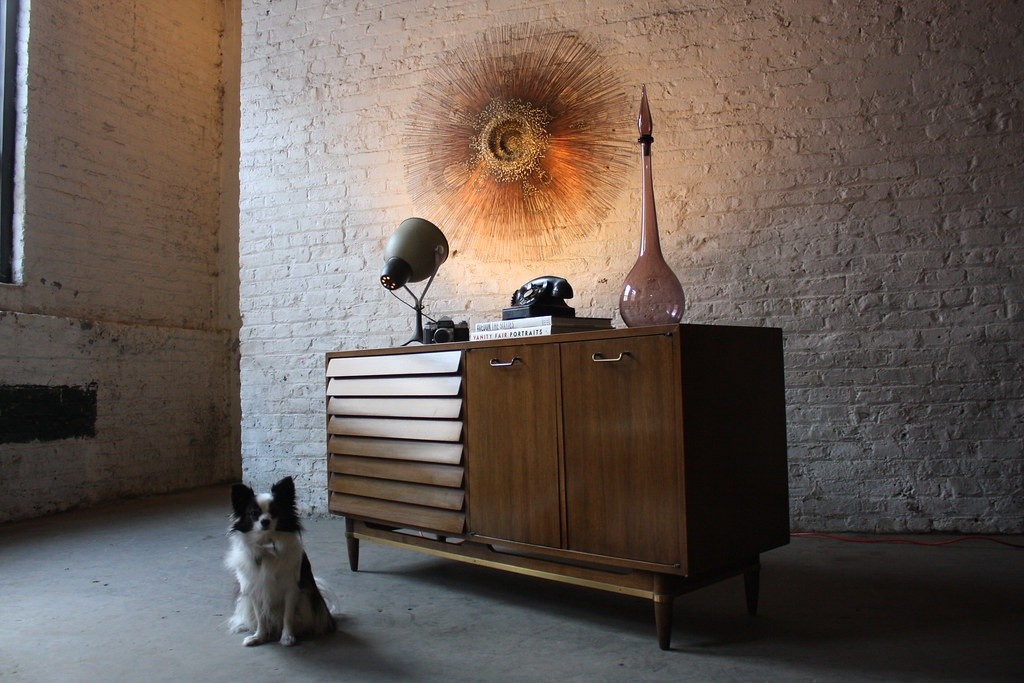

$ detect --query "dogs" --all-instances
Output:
[226,475,340,648]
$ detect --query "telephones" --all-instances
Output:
[501,275,575,320]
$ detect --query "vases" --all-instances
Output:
[618,84,685,327]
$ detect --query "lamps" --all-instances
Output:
[372,213,452,346]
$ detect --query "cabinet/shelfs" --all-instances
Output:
[319,322,796,655]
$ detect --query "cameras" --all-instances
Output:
[423,317,470,344]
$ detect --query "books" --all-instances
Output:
[471,315,616,341]
